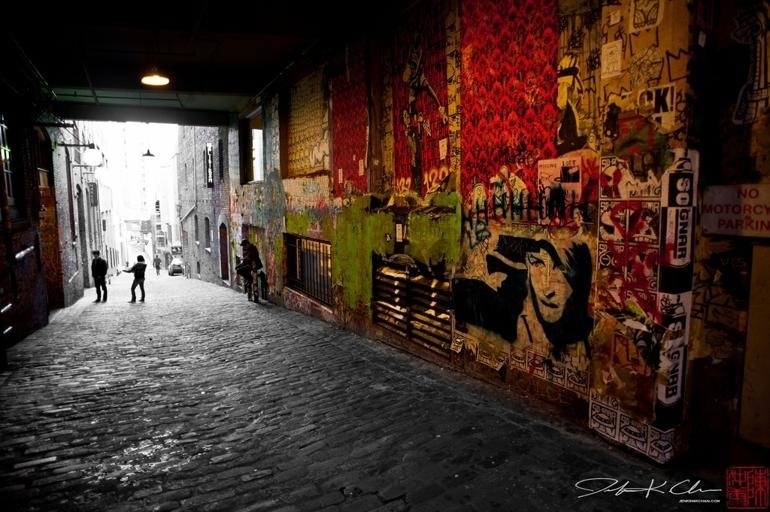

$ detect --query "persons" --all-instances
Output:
[92,250,108,302]
[122,255,147,303]
[235,239,259,287]
[517,236,596,367]
[398,42,447,193]
[153,255,161,275]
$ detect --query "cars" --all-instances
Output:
[167,257,182,277]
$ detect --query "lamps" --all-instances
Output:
[142,149,155,157]
[141,67,170,86]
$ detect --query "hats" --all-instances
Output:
[240,240,250,245]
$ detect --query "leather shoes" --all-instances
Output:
[128,299,144,303]
[244,280,253,287]
